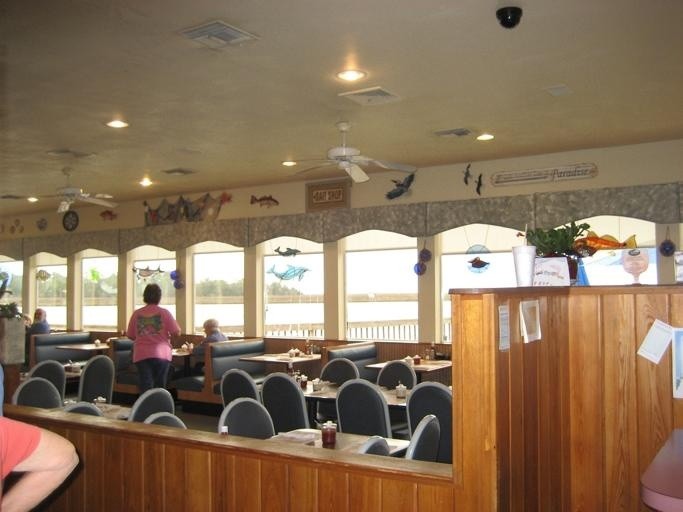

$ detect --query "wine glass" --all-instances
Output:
[622,251,649,285]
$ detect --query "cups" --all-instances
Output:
[289,348,300,357]
[300,374,323,392]
[322,420,337,445]
[673,249,683,283]
[95,339,100,347]
[181,342,195,352]
[396,384,406,399]
[404,354,421,365]
[513,246,537,287]
[71,363,81,372]
[93,396,106,414]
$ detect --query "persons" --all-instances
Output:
[24,308,49,335]
[178,320,230,378]
[127,284,181,395]
[0,415,80,512]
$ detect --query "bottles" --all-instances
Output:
[305,338,311,354]
[220,426,229,435]
[288,360,294,375]
[425,341,436,360]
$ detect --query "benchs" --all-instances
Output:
[169,339,260,402]
[29,331,90,390]
[320,342,380,384]
[109,339,140,395]
[209,338,276,403]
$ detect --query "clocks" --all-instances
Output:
[63,211,79,231]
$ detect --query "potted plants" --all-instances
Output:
[526,219,591,284]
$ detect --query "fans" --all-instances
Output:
[31,168,120,214]
[281,121,418,183]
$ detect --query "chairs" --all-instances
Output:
[127,388,176,423]
[11,377,63,409]
[377,360,417,431]
[405,414,440,462]
[75,354,115,404]
[407,382,452,465]
[262,372,310,432]
[360,435,390,457]
[28,359,67,400]
[218,397,275,440]
[143,411,186,429]
[316,358,359,432]
[335,379,411,441]
[63,401,102,416]
[221,368,261,405]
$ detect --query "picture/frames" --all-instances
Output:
[305,179,352,213]
